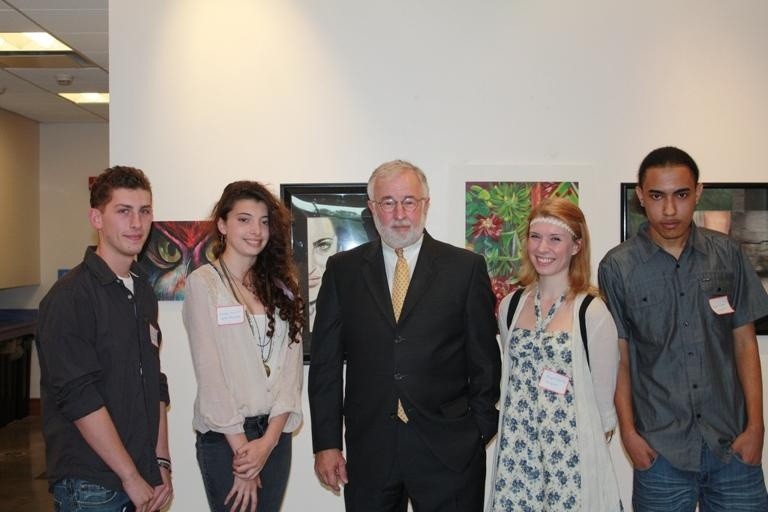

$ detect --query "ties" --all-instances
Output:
[391,247,413,426]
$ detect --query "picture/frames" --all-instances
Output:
[276,181,402,367]
[615,178,768,337]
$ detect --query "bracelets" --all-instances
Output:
[157,456,171,474]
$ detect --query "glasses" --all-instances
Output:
[369,197,429,213]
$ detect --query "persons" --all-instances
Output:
[597,147,768,511]
[484,198,634,511]
[307,161,502,512]
[36,166,173,512]
[181,181,308,512]
[307,209,369,333]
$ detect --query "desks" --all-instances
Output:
[0,308,40,432]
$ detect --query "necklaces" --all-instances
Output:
[218,253,275,378]
